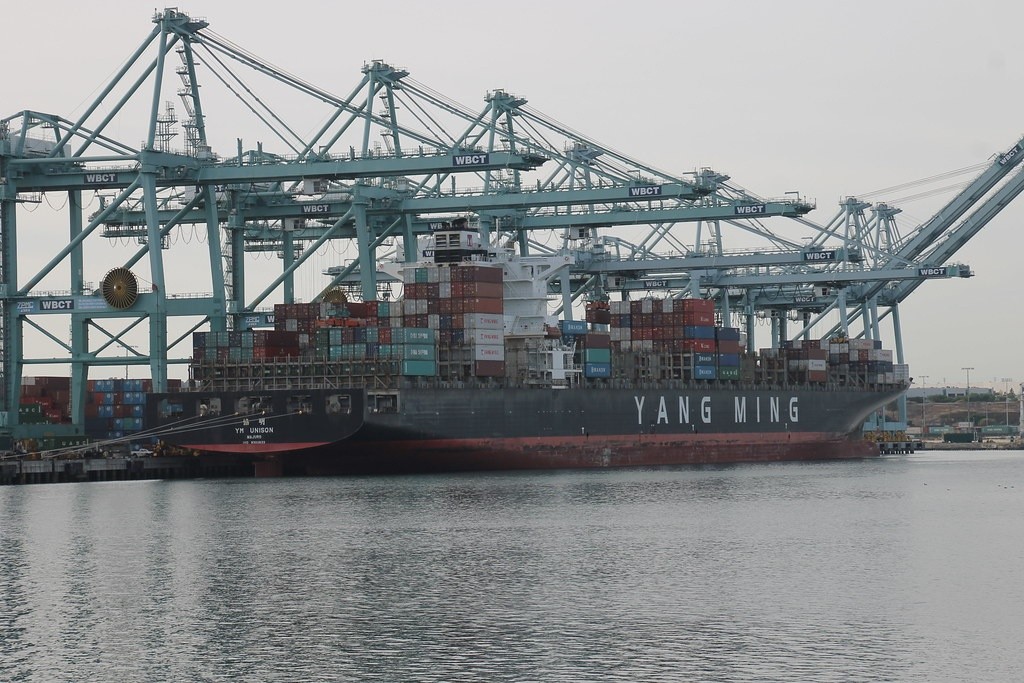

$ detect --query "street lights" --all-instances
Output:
[918,376,930,428]
[116,345,139,379]
[1001,377,1012,427]
[961,367,974,428]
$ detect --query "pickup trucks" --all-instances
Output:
[131,449,153,458]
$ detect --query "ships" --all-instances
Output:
[140,267,1024,487]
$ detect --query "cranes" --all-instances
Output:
[0,2,1024,445]
